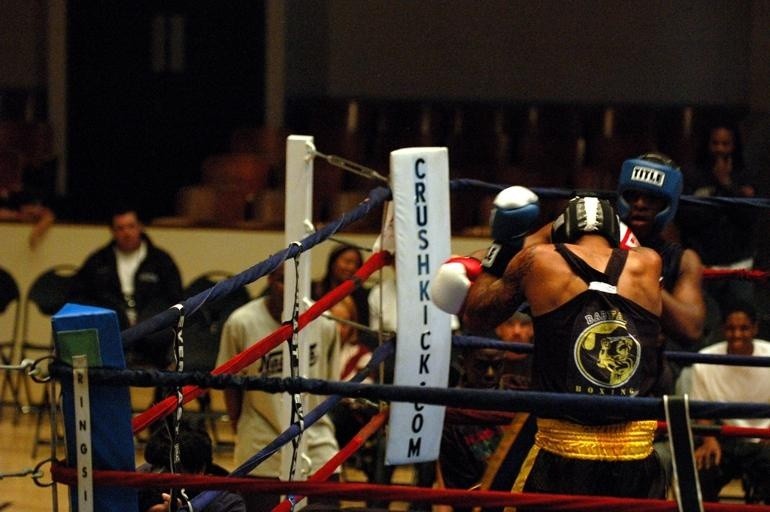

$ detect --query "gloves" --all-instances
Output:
[482,186,541,274]
[620,222,642,249]
[430,257,482,316]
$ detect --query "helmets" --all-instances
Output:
[618,153,684,238]
[552,191,619,247]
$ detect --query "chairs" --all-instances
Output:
[182,269,252,322]
[0,262,80,424]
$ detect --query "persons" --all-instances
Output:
[134,428,244,511]
[440,303,533,511]
[436,153,704,392]
[74,211,182,368]
[468,193,667,511]
[216,259,342,510]
[311,250,435,511]
[674,297,770,505]
[0,126,770,250]
[181,269,251,409]
